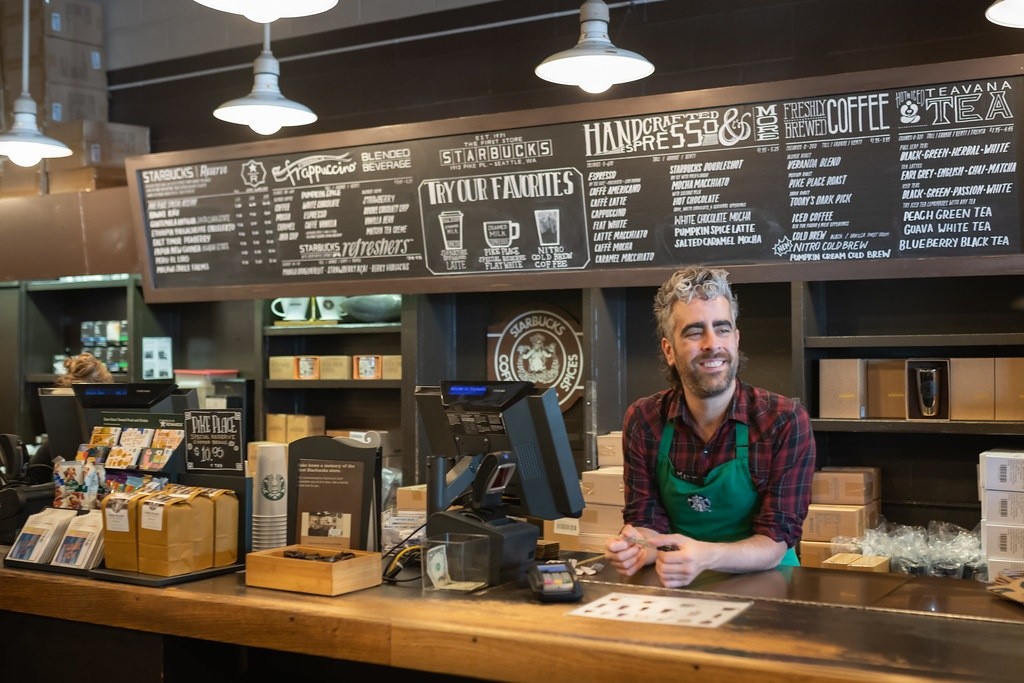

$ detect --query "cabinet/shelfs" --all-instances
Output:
[0,277,1024,621]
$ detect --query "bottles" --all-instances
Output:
[81,320,128,372]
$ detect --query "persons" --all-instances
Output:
[605,266,816,588]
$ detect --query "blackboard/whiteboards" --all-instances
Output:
[126,54,1024,302]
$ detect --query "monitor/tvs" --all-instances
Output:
[415,380,586,521]
[37,380,200,467]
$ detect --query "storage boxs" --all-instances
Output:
[246,349,1024,597]
[0,0,150,203]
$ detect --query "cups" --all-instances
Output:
[315,296,348,320]
[253,445,288,552]
[271,298,310,321]
[299,359,315,377]
[360,357,375,378]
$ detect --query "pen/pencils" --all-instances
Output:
[618,535,677,553]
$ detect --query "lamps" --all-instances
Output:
[213,24,318,135]
[534,0,656,94]
[0,0,75,170]
